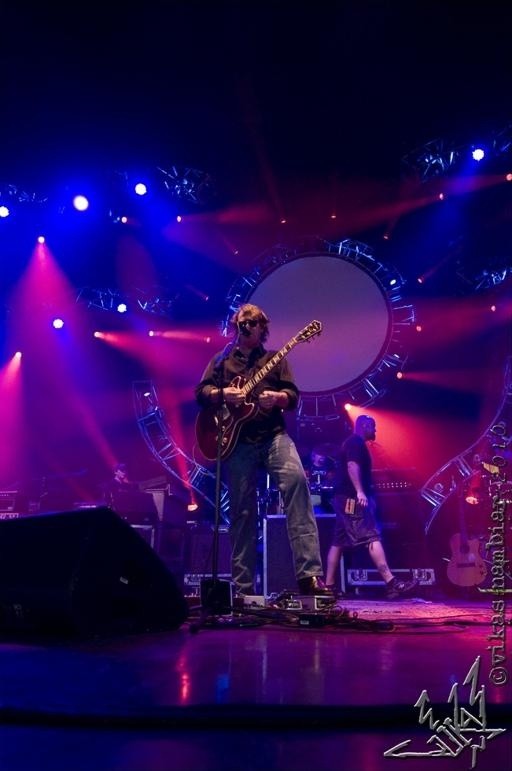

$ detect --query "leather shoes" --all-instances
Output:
[298,576,335,595]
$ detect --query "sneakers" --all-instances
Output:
[385,578,419,599]
[326,584,351,600]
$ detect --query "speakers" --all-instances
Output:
[0,505,188,645]
[189,526,232,578]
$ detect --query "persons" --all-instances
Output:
[102,464,133,508]
[190,302,345,619]
[301,446,335,496]
[321,414,421,603]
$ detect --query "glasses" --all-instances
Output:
[238,319,259,327]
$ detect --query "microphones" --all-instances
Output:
[238,321,251,338]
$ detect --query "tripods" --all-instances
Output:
[185,336,291,634]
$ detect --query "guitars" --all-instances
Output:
[447,479,486,587]
[195,320,322,460]
